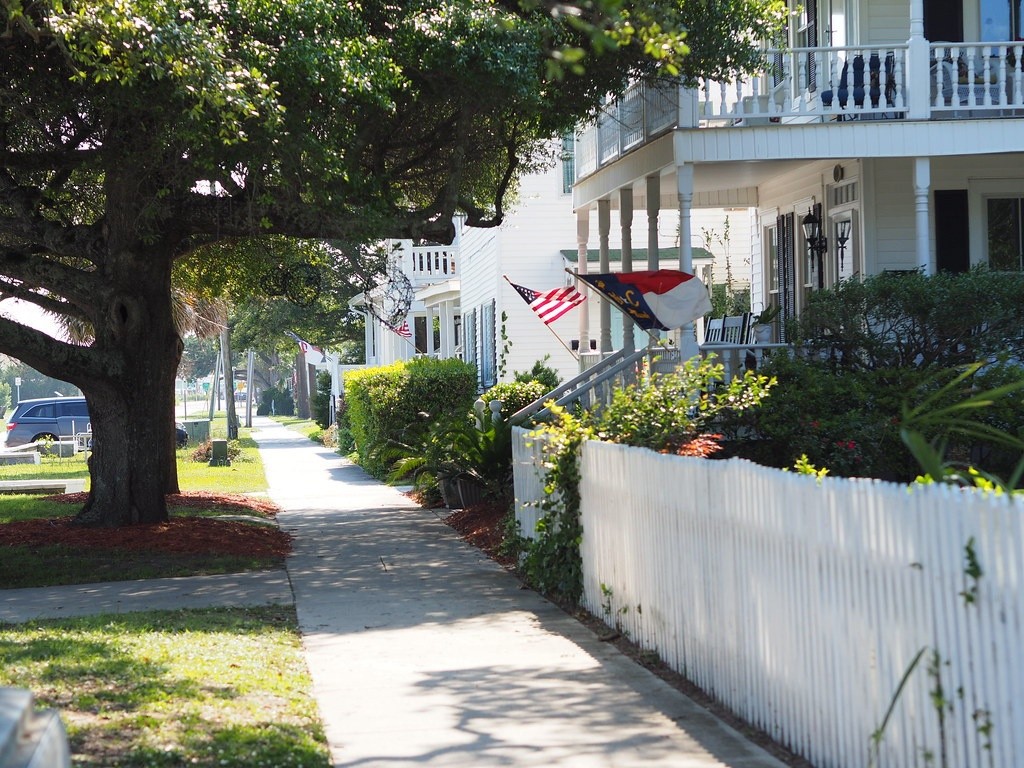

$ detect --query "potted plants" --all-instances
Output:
[750,302,783,344]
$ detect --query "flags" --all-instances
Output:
[574,269,714,330]
[284,329,327,367]
[510,283,587,325]
[392,320,412,338]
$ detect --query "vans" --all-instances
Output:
[6,397,188,458]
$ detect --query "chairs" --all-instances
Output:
[929,41,1006,118]
[732,75,792,126]
[721,313,748,344]
[820,51,899,119]
[744,312,763,345]
[704,315,727,342]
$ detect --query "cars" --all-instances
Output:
[234,388,247,401]
